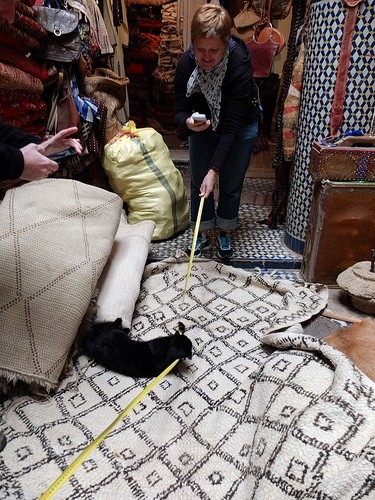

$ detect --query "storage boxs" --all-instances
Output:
[301,179,375,284]
[300,136,375,181]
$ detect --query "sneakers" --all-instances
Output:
[185,235,212,258]
[216,232,234,259]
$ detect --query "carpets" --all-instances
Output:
[0,0,375,500]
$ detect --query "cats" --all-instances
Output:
[73,318,192,379]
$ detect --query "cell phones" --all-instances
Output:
[192,114,206,124]
[46,147,78,162]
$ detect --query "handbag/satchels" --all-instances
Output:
[231,0,261,44]
[31,6,81,62]
[244,21,285,77]
[40,62,108,179]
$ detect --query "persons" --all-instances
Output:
[0,127,83,182]
[172,4,263,259]
